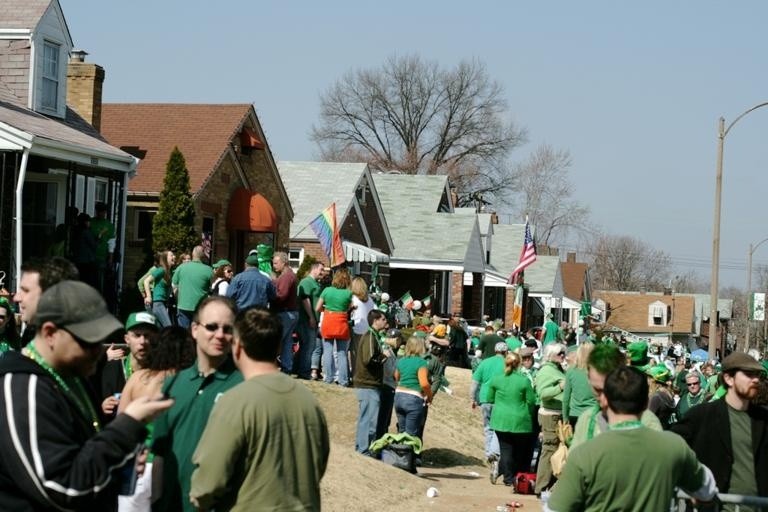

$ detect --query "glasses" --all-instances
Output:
[0,315,9,319]
[223,269,232,273]
[686,382,699,386]
[194,319,233,334]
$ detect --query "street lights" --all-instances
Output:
[667,274,679,291]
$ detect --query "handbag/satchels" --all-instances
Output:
[512,472,538,494]
[380,443,417,474]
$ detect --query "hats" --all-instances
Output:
[125,311,162,334]
[626,341,650,363]
[35,280,125,346]
[721,352,768,374]
[494,342,508,352]
[211,258,231,269]
[385,328,402,338]
[249,249,257,256]
[643,363,671,384]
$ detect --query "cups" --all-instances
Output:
[118,430,146,496]
[111,393,122,416]
[426,487,438,497]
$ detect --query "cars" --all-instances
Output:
[523,326,542,342]
[435,317,486,334]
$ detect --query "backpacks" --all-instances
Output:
[207,279,230,297]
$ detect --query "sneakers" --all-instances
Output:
[490,460,500,484]
[310,373,319,380]
[416,457,423,466]
[487,454,500,463]
